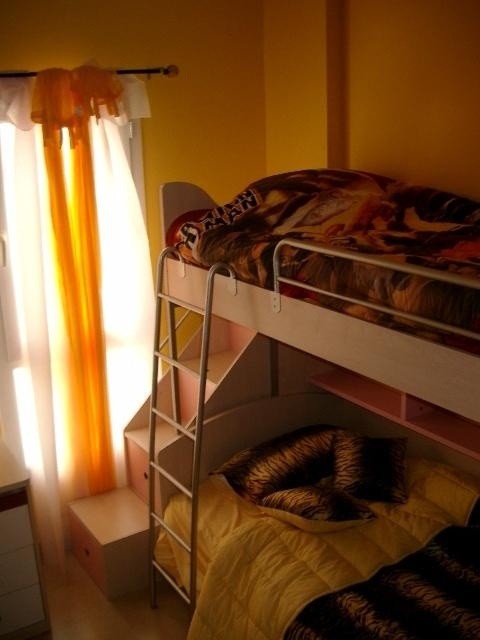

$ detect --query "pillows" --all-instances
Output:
[209,420,408,535]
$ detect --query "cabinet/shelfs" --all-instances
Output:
[0,436,51,640]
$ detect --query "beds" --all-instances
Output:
[148,179,480,639]
